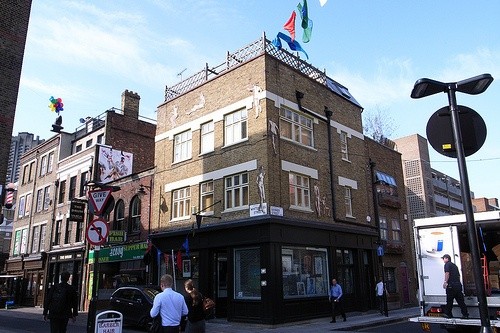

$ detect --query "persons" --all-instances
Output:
[329,278,346,323]
[183,279,206,333]
[441,254,469,319]
[43,272,78,333]
[150,274,188,333]
[376,280,390,314]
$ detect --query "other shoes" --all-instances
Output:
[330,320,337,323]
[460,315,469,319]
[343,316,346,322]
[443,314,453,318]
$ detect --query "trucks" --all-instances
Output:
[407,212,500,330]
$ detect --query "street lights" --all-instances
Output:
[409,72,496,333]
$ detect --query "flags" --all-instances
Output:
[271,36,281,51]
[284,11,296,43]
[302,19,313,43]
[297,0,308,30]
[277,32,309,59]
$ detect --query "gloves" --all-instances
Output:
[442,281,448,289]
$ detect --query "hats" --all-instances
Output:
[441,254,451,258]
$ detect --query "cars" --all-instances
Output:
[108,286,189,333]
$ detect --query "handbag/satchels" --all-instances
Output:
[202,297,216,311]
